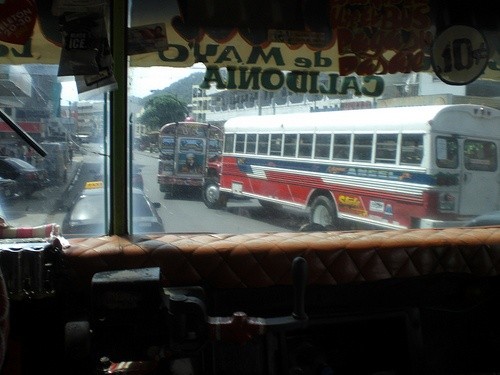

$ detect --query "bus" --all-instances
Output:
[202,104,500,230]
[157,122,224,197]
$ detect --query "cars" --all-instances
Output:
[62,181,165,239]
[0,155,50,197]
[0,177,24,207]
[90,161,144,189]
